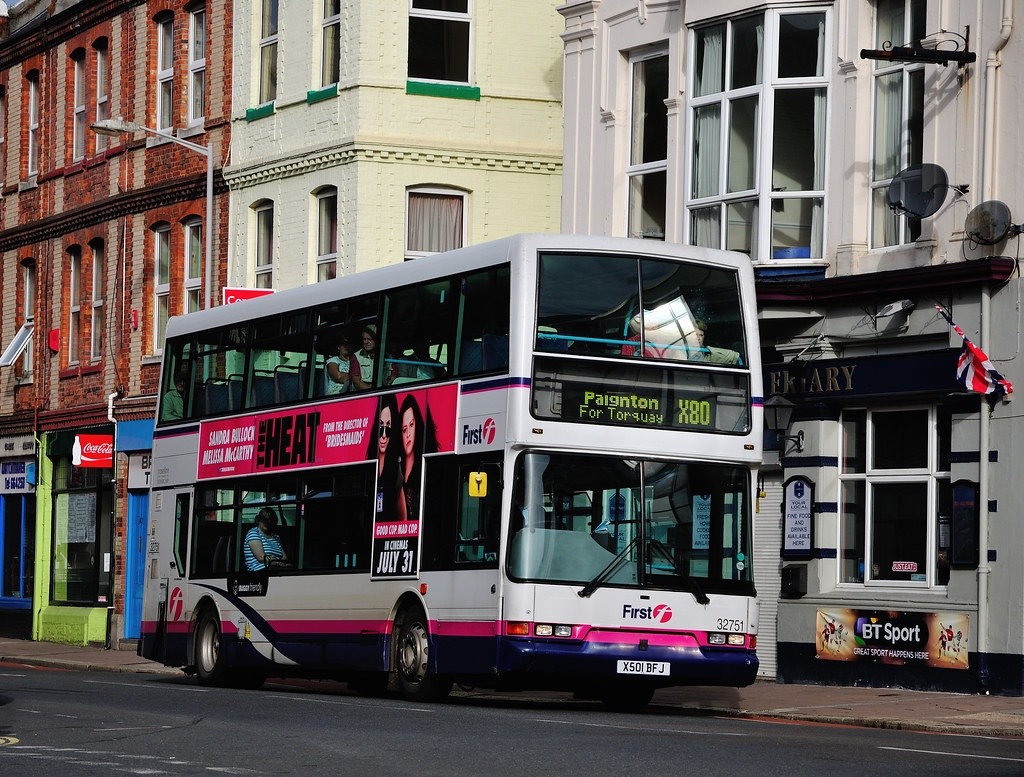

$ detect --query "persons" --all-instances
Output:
[622,303,669,359]
[243,507,299,577]
[934,623,966,661]
[161,371,188,421]
[365,394,408,525]
[325,336,354,395]
[349,324,397,392]
[399,396,439,520]
[820,617,848,652]
[673,319,740,364]
[398,330,443,383]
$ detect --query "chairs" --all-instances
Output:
[192,326,567,576]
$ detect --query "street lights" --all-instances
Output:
[88,118,215,386]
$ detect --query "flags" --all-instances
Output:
[938,307,1012,410]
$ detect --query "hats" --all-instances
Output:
[338,335,352,343]
[174,371,187,385]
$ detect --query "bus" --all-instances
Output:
[138,231,766,716]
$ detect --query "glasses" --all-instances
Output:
[378,426,391,438]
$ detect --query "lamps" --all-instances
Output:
[763,392,805,452]
